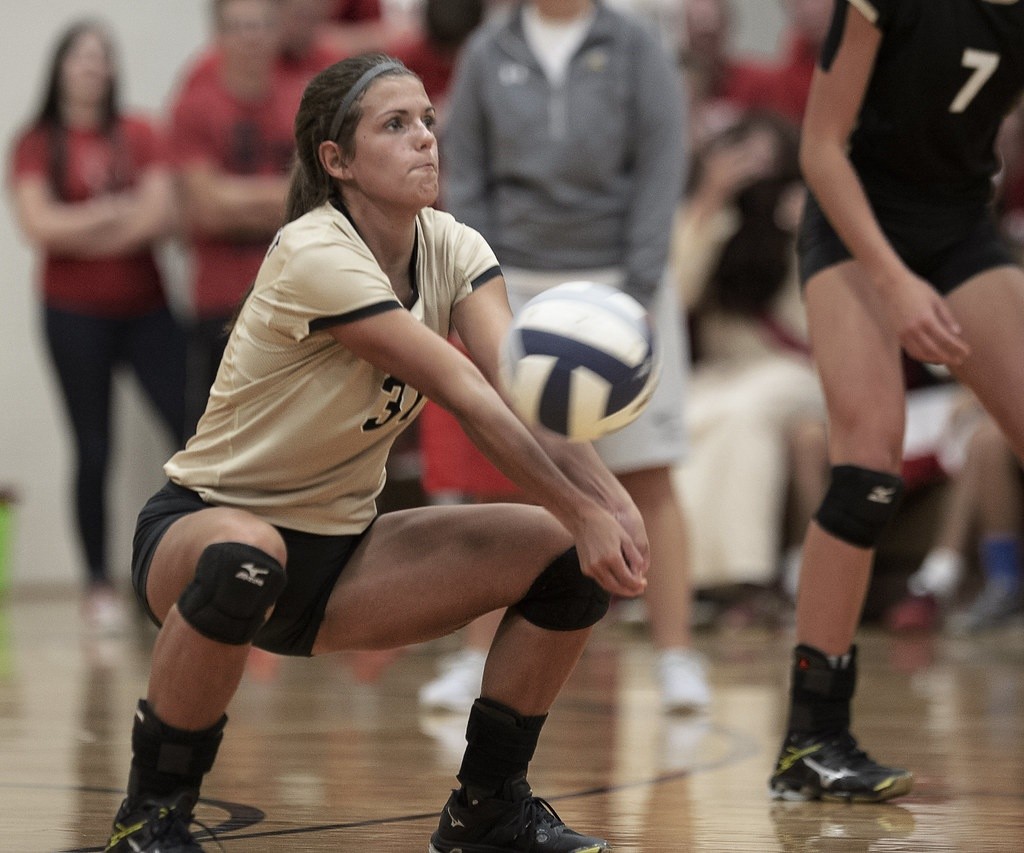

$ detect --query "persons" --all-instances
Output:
[767,0,1024,804]
[0,0,1024,714]
[105,53,652,853]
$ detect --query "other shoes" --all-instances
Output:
[418,650,485,710]
[971,581,1024,621]
[655,647,711,712]
[907,551,970,598]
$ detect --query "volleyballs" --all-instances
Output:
[497,279,665,445]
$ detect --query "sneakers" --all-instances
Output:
[767,729,911,805]
[428,778,610,853]
[104,800,226,853]
